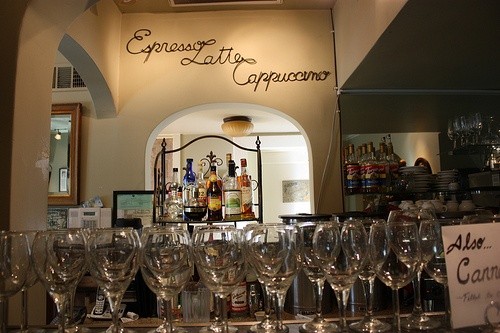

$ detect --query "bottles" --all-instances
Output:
[164,155,252,221]
[230,263,248,317]
[342,134,399,192]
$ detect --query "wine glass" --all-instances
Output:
[0,213,500,333]
[447,111,500,149]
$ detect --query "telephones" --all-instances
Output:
[90,285,127,320]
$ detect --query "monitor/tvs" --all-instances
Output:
[59,168,68,192]
[112,191,170,228]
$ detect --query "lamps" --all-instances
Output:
[222,116,255,139]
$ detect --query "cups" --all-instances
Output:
[389,200,475,212]
[181,282,210,322]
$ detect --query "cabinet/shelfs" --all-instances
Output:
[337,92,499,313]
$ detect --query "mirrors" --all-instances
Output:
[47,102,82,207]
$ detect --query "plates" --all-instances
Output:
[400,167,459,192]
